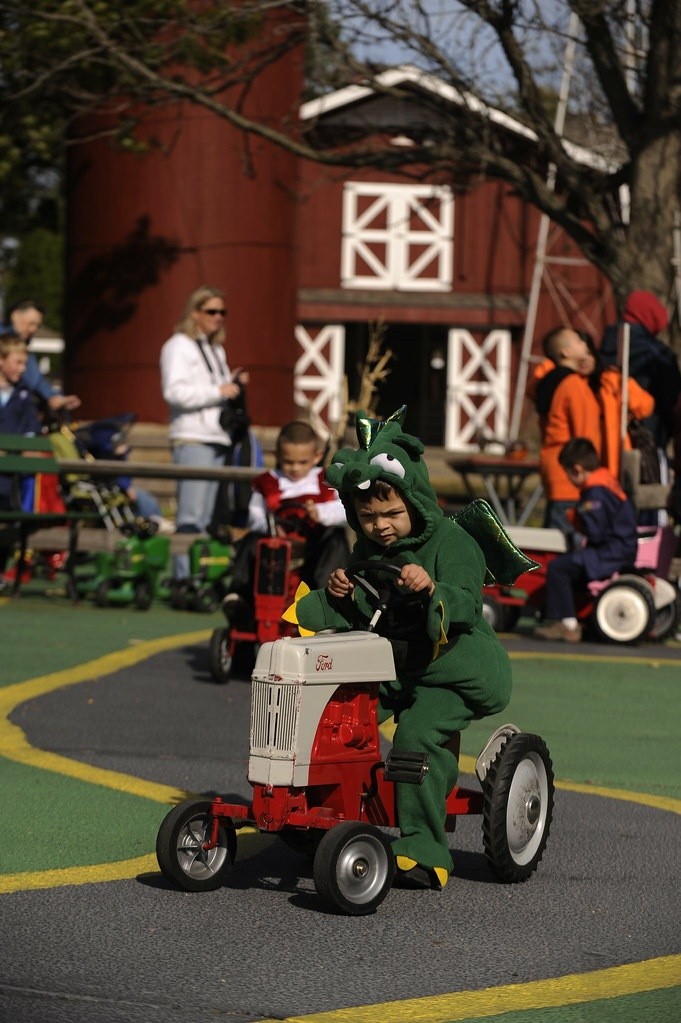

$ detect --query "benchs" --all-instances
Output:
[1,435,106,601]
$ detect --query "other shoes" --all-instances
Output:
[391,858,436,887]
[534,619,583,642]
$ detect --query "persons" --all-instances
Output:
[160,285,249,587]
[222,422,352,623]
[0,299,176,593]
[527,289,681,644]
[282,404,542,891]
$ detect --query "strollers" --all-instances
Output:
[39,406,177,570]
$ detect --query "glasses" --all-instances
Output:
[197,308,226,316]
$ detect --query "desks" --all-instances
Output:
[452,453,546,525]
